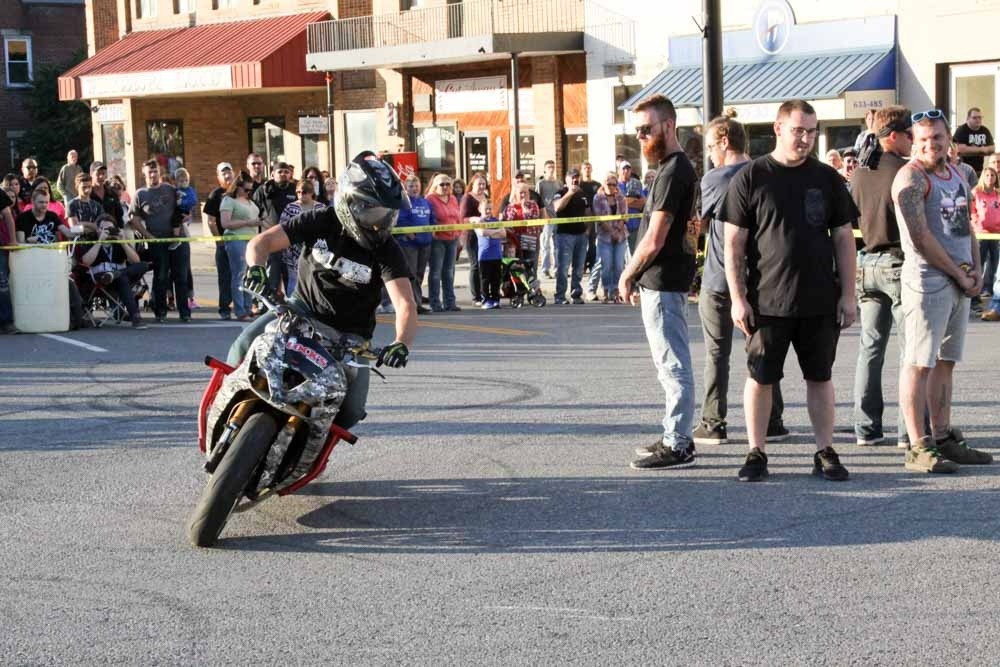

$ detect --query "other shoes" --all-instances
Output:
[102,272,113,286]
[240,315,255,321]
[168,242,182,251]
[133,320,149,330]
[981,288,991,296]
[221,312,229,320]
[182,313,192,322]
[970,302,983,312]
[158,315,169,323]
[413,287,640,313]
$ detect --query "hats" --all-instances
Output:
[91,162,107,171]
[217,162,233,171]
[567,169,579,177]
[514,171,524,178]
[620,161,631,170]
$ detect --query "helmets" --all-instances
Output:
[333,151,411,251]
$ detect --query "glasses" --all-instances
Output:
[779,124,820,138]
[438,183,450,188]
[636,118,668,135]
[705,139,722,152]
[242,186,254,192]
[23,166,38,170]
[912,109,948,130]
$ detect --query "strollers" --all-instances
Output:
[498,227,547,309]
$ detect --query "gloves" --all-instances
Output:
[242,267,271,300]
[375,341,409,370]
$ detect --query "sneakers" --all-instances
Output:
[630,418,993,481]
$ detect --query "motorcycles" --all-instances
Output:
[186,270,408,547]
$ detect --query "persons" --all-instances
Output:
[0,96,999,482]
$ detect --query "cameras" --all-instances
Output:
[104,227,118,236]
